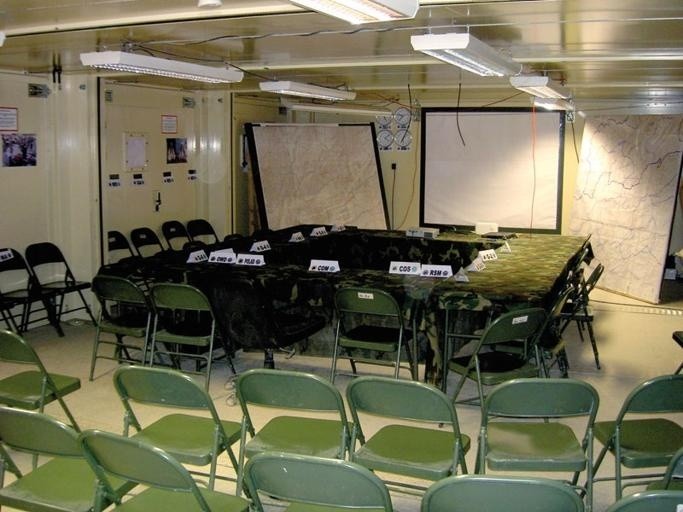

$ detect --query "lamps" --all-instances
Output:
[289,0,419,24]
[532,96,575,111]
[409,32,523,78]
[79,49,245,85]
[257,79,357,103]
[508,75,572,100]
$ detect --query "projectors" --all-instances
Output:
[406,226,440,239]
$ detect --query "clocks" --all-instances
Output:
[376,129,394,147]
[394,129,413,148]
[393,106,411,124]
[374,108,393,127]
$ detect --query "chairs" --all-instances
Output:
[1,247,62,340]
[24,241,99,338]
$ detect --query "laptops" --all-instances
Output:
[481,231,515,239]
